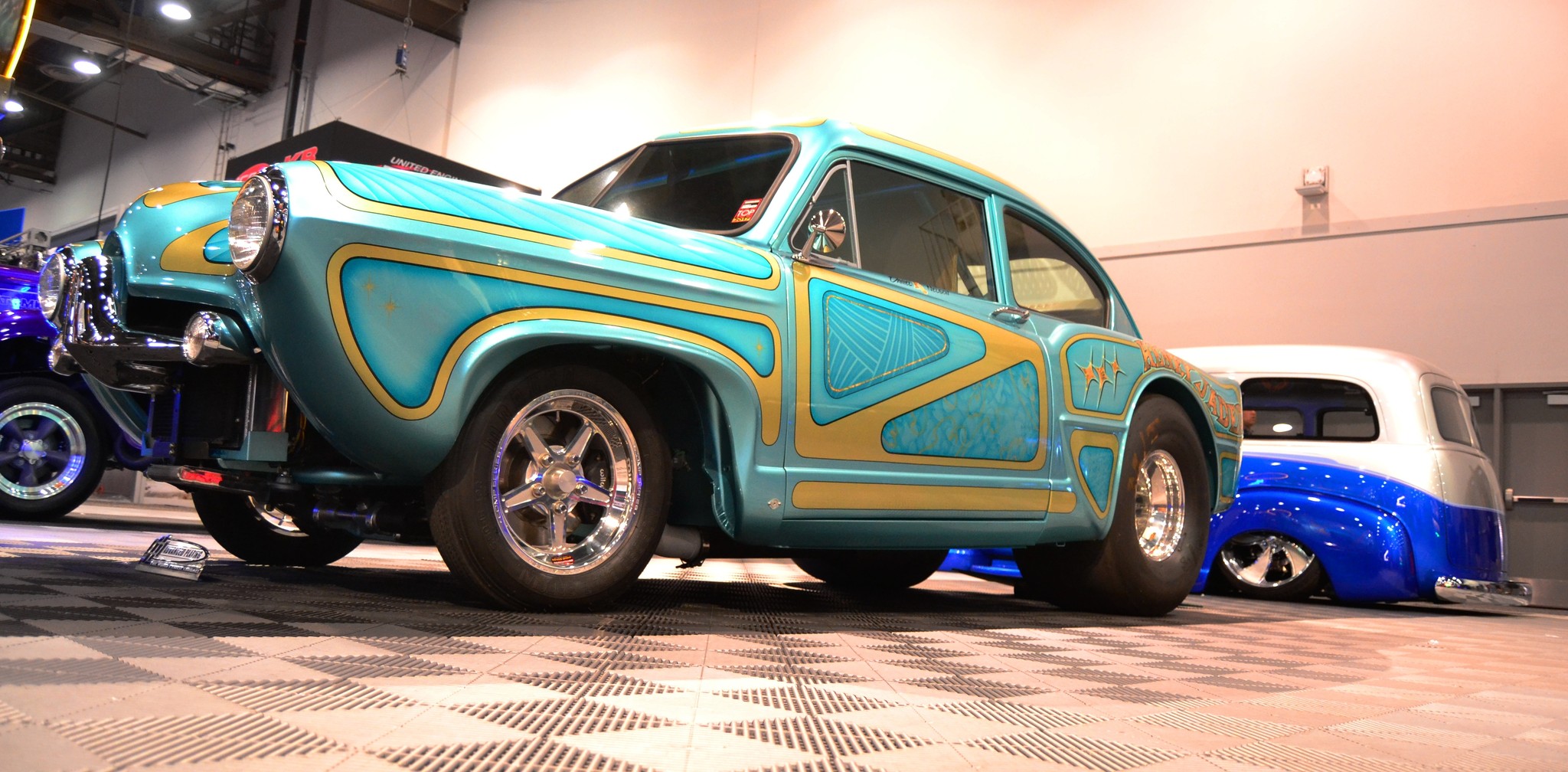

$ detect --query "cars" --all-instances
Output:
[940,344,1531,611]
[38,122,1245,628]
[0,226,183,524]
